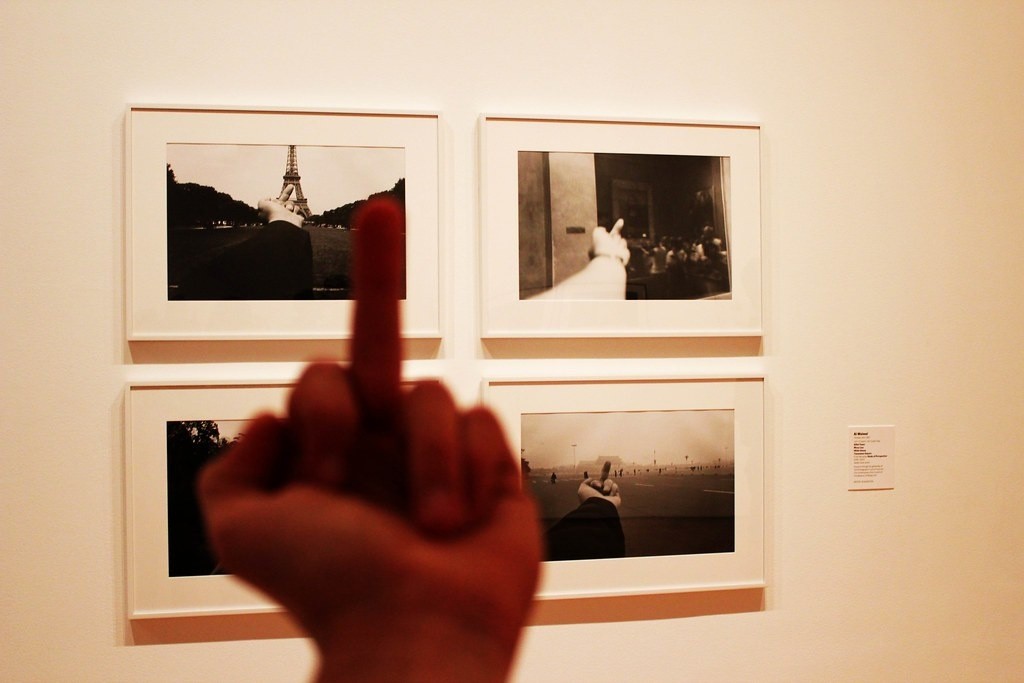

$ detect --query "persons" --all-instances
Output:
[542,461,626,561]
[551,471,557,484]
[628,225,725,272]
[180,185,314,301]
[200,199,542,683]
[525,220,631,300]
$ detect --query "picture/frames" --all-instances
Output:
[125,100,445,343]
[121,374,451,620]
[480,374,772,602]
[476,114,765,340]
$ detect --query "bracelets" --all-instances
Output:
[591,251,626,264]
